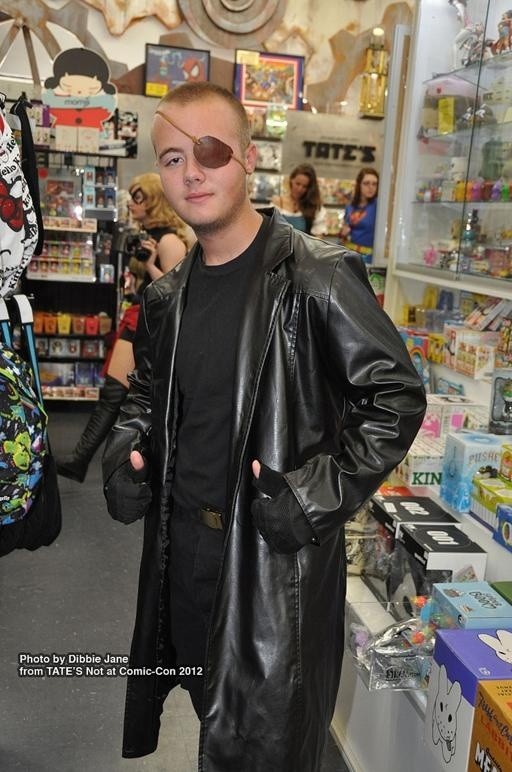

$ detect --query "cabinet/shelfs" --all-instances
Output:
[328,0,512,772]
[248,136,350,240]
[26,109,119,410]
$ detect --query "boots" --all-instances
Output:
[55,373,128,482]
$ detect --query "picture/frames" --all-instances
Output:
[144,43,210,99]
[235,49,303,111]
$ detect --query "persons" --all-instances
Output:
[271,163,320,233]
[102,82,426,769]
[40,47,116,155]
[490,10,512,52]
[339,168,377,264]
[51,174,186,483]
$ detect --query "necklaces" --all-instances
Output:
[293,197,301,214]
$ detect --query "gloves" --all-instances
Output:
[249,462,314,554]
[104,461,152,524]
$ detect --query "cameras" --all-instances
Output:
[127,228,150,262]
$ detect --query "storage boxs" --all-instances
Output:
[440,433,502,513]
[388,525,488,623]
[468,679,512,772]
[423,628,512,772]
[423,581,512,684]
[394,436,445,487]
[360,497,460,608]
